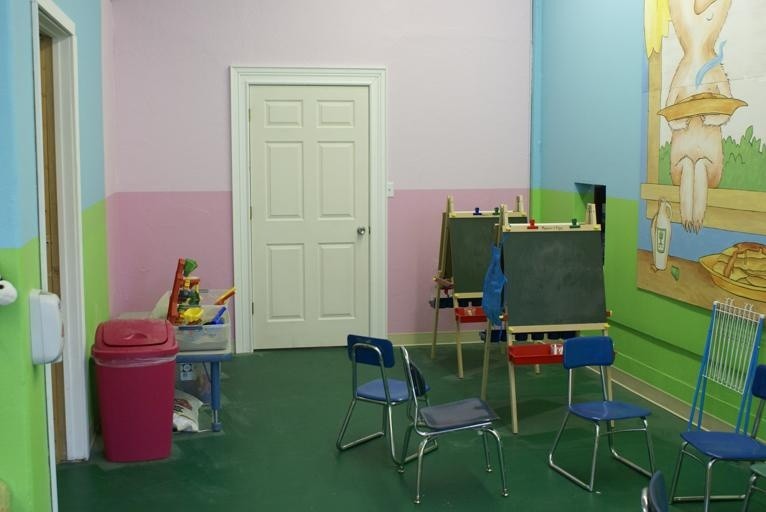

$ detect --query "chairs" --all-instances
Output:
[397,346,510,505]
[670,364,766,511]
[548,335,655,494]
[336,334,438,464]
[640,467,667,511]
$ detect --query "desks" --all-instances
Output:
[176,351,227,433]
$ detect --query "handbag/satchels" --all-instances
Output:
[172,388,204,433]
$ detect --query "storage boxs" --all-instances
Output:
[172,306,232,352]
[178,362,222,407]
[197,287,230,310]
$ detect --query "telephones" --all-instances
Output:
[29,289,64,364]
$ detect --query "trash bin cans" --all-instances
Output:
[91,318,179,462]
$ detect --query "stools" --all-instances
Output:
[741,460,765,512]
